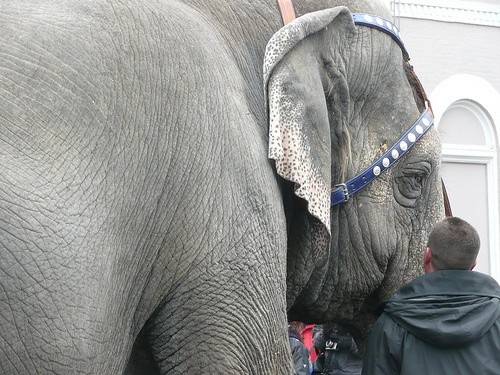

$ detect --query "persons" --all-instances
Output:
[361,217,500,375]
[287,322,366,375]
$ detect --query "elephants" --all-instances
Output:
[0,1,447,375]
